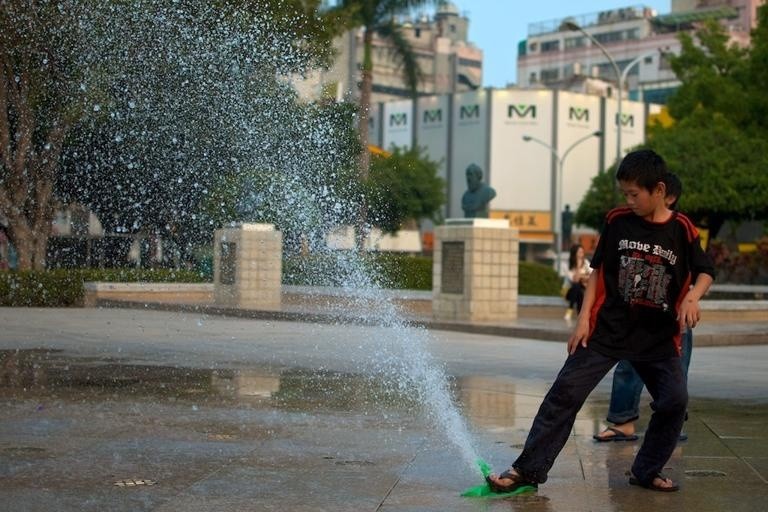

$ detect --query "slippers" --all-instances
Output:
[593,426,638,442]
[629,473,679,492]
[486,470,538,493]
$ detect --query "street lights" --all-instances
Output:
[563,17,710,209]
[522,131,605,272]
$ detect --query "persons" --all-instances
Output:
[562,205,575,252]
[486,147,720,495]
[591,174,698,443]
[561,245,593,331]
[460,163,497,211]
